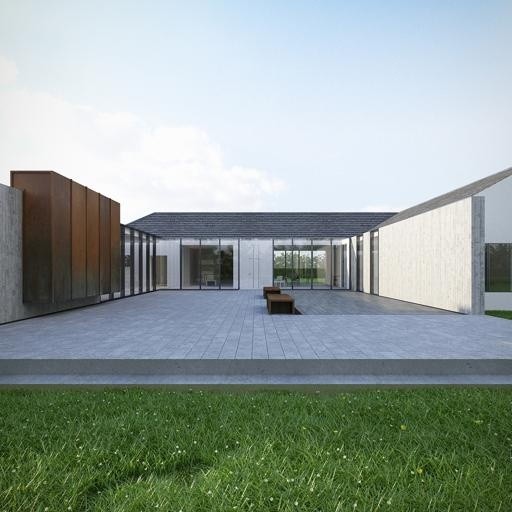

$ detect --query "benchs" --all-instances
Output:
[263,286,281,298]
[266,292,296,315]
